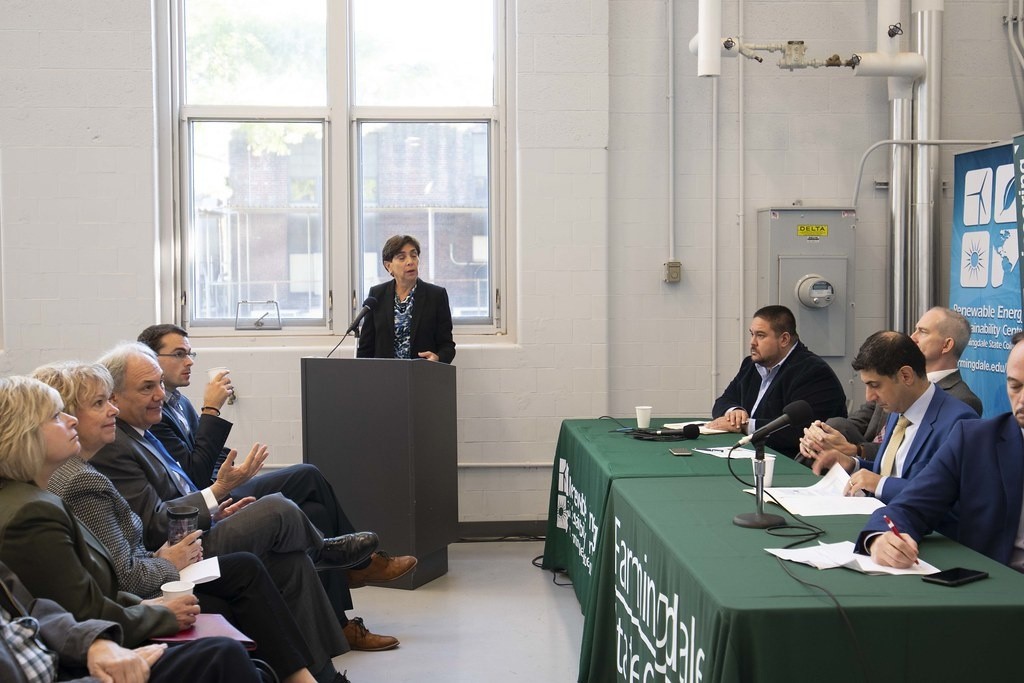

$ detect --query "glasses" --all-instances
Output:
[157,350,197,361]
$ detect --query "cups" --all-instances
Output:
[167,506,200,548]
[750,456,776,488]
[635,406,653,428]
[160,580,195,602]
[205,367,228,384]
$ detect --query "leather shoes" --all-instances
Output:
[314,531,379,572]
[347,551,418,589]
[335,670,351,683]
[343,617,399,651]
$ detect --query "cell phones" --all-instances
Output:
[921,567,990,586]
[669,448,692,456]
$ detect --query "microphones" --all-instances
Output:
[733,399,814,449]
[346,297,378,334]
[648,424,700,440]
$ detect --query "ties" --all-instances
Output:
[143,430,198,492]
[880,415,911,478]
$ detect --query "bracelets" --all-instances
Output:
[200,405,220,416]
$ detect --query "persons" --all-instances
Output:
[19,358,320,683]
[853,331,1023,575]
[705,305,849,460]
[352,234,458,364]
[0,374,268,683]
[796,305,984,477]
[136,321,419,652]
[86,339,378,683]
[809,329,979,504]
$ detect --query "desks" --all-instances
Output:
[542,418,1024,683]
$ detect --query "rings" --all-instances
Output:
[849,480,853,486]
[716,423,718,425]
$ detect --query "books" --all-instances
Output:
[669,448,692,456]
[662,419,730,435]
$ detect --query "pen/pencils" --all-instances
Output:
[883,515,920,566]
